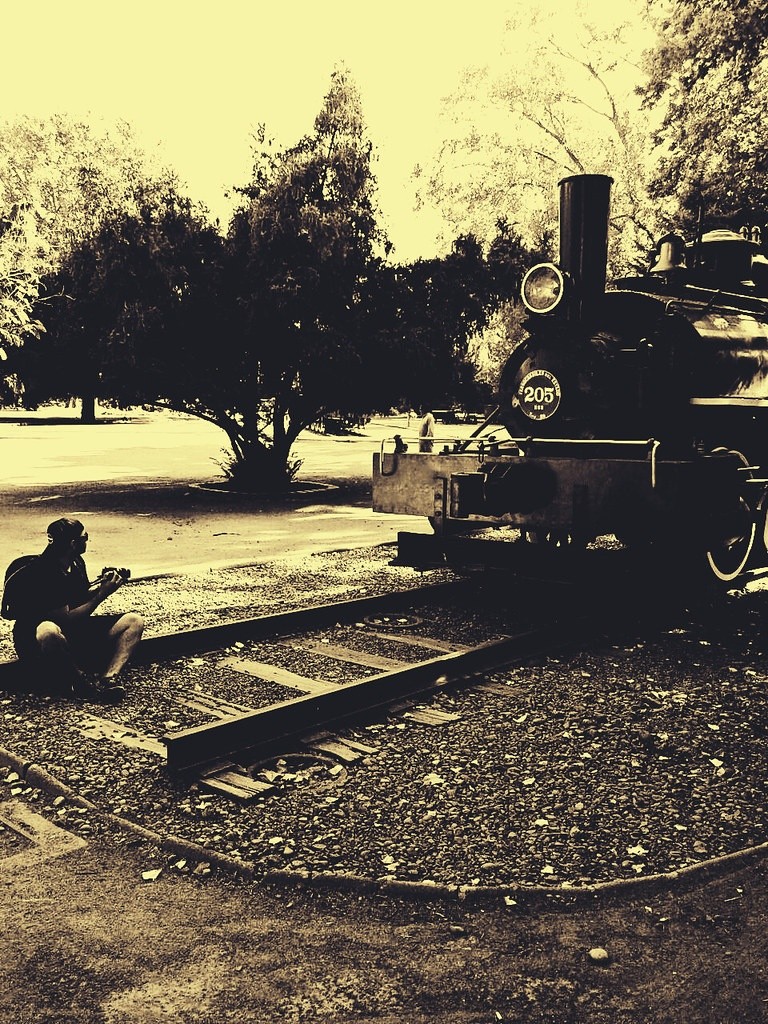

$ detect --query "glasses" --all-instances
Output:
[80,532,88,540]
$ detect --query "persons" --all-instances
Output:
[12,516,145,698]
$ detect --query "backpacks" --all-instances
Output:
[1,553,44,620]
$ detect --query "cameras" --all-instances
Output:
[99,567,131,581]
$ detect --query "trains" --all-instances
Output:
[370,174,768,597]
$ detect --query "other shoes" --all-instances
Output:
[99,676,126,700]
[66,670,97,701]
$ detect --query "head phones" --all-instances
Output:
[59,518,77,551]
